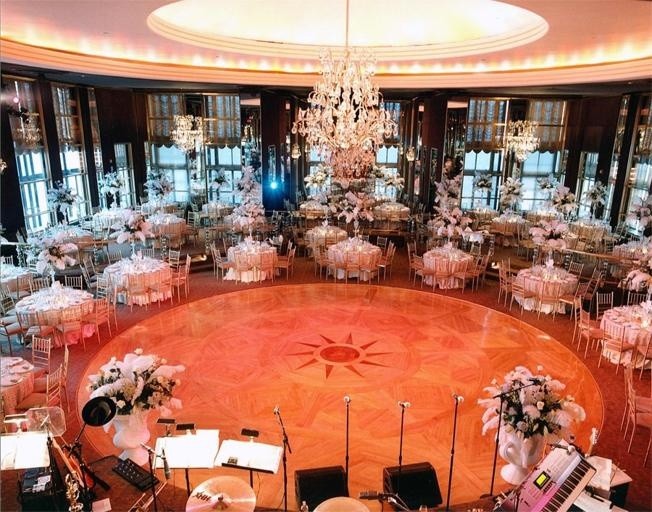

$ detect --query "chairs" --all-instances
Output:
[2,204,95,469]
[555,218,650,466]
[96,194,554,320]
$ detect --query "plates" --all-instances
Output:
[519,268,578,283]
[19,293,94,312]
[0,356,34,388]
[604,306,636,329]
[104,253,167,278]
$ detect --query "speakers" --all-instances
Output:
[294,465,349,512]
[382,462,443,512]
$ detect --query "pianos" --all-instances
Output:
[492,437,597,512]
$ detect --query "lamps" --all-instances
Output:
[169,88,210,154]
[503,93,541,166]
[290,0,400,170]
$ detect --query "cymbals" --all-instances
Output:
[185,475,256,512]
[312,497,371,512]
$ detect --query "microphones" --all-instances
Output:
[549,443,576,453]
[358,490,384,500]
[452,392,464,402]
[527,377,544,383]
[398,400,410,408]
[343,396,351,404]
[273,404,279,415]
[39,414,50,429]
[162,447,171,480]
